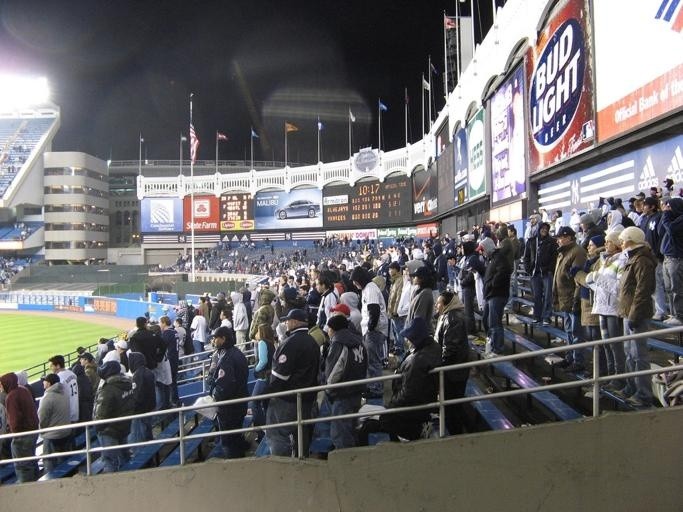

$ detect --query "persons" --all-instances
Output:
[1,144,683,483]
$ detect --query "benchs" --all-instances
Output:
[2,116,58,240]
[3,240,683,485]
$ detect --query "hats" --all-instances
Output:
[604,226,645,244]
[475,246,483,251]
[636,191,645,197]
[279,308,309,323]
[76,352,93,363]
[327,315,349,331]
[207,326,234,340]
[329,304,350,316]
[551,226,576,238]
[112,340,128,352]
[574,213,594,225]
[40,373,61,384]
[650,187,659,193]
[408,267,433,278]
[283,287,300,300]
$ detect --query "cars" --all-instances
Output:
[273,197,321,220]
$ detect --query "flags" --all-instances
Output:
[405,96,411,103]
[318,121,323,131]
[379,101,388,112]
[218,133,228,140]
[181,136,187,142]
[286,123,300,132]
[431,62,441,77]
[423,79,429,91]
[141,137,144,142]
[189,121,200,163]
[252,130,259,138]
[445,14,459,29]
[350,111,356,123]
